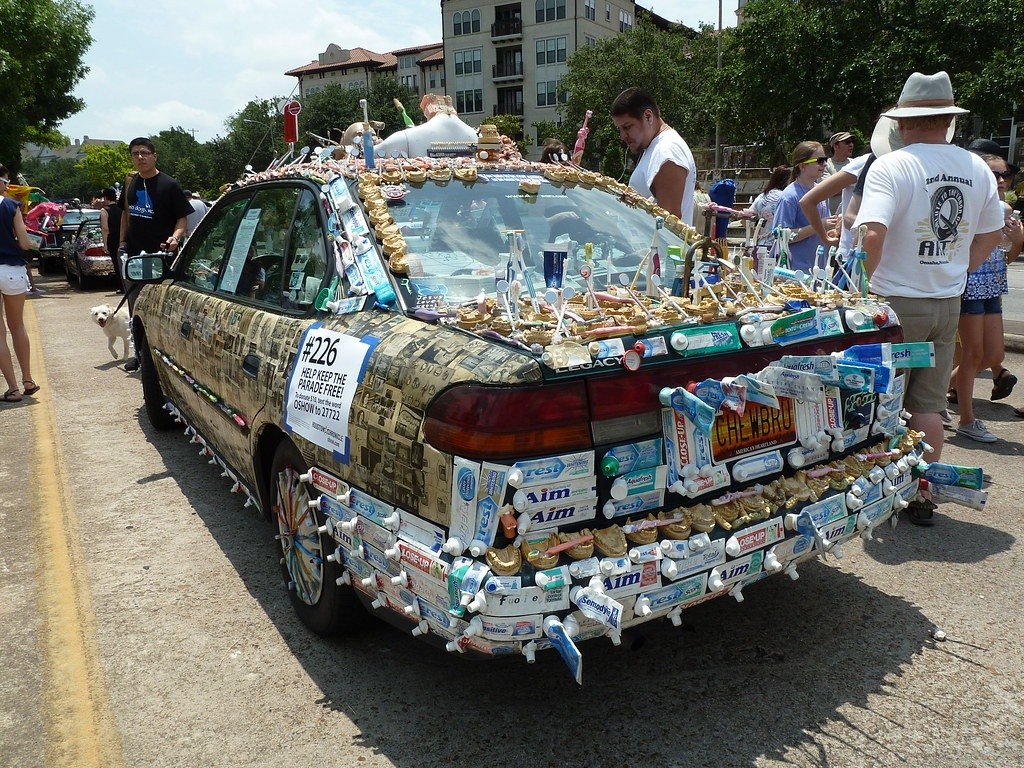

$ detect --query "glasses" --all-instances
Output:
[842,140,855,145]
[992,171,1012,181]
[131,150,152,158]
[0,178,10,185]
[794,156,828,167]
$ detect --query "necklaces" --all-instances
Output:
[987,254,1009,281]
[795,176,824,219]
[654,125,669,136]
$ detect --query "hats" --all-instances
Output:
[192,193,200,198]
[967,139,1004,158]
[1006,162,1021,176]
[869,106,956,159]
[880,71,970,118]
[100,189,116,195]
[829,132,855,148]
[183,190,192,196]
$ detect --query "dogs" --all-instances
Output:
[90,303,133,362]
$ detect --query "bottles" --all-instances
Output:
[997,209,1021,252]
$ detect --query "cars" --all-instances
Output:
[124,161,931,666]
[60,217,119,287]
[38,209,103,274]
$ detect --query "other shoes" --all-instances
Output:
[116,289,125,295]
[30,288,37,292]
[901,496,938,525]
[125,358,140,370]
[1014,407,1024,415]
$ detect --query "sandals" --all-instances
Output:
[0,389,22,402]
[947,388,958,404]
[23,380,40,395]
[990,368,1017,400]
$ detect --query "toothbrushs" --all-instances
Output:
[850,225,867,293]
[823,245,837,290]
[648,216,664,296]
[506,231,514,300]
[772,228,791,269]
[517,236,541,315]
[584,242,594,308]
[694,249,703,305]
[809,244,824,291]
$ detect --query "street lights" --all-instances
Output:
[243,119,275,160]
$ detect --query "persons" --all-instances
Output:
[100,137,212,371]
[740,71,1023,526]
[609,87,718,254]
[539,107,593,166]
[0,163,41,402]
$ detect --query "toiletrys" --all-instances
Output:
[162,401,262,512]
[298,304,989,686]
[543,243,568,304]
[741,247,776,288]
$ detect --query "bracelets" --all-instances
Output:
[120,240,127,244]
[173,236,181,242]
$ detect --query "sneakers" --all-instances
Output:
[937,396,952,422]
[955,419,997,442]
